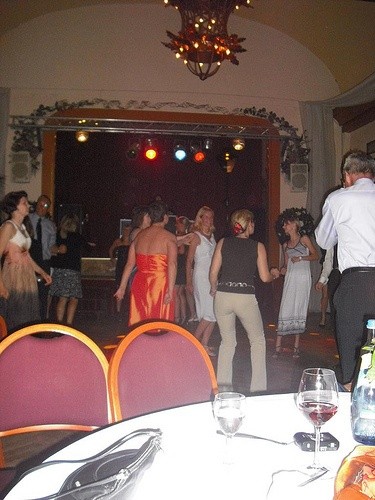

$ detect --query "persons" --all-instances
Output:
[185,206,218,356]
[113,200,178,329]
[22,195,198,327]
[0,190,52,335]
[315,245,340,326]
[210,209,279,393]
[315,150,375,384]
[273,215,319,359]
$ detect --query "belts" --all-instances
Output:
[340,267,375,278]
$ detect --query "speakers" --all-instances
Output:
[289,163,309,192]
[11,149,31,184]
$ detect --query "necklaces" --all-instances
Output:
[203,231,210,236]
[12,218,22,230]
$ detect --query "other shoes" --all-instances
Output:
[203,344,216,357]
[319,322,325,328]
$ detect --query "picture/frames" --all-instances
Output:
[165,215,178,237]
[118,218,132,238]
[57,202,83,237]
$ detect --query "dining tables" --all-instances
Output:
[2,391,375,500]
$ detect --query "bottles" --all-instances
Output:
[351,320,375,445]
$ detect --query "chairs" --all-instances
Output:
[0,323,112,496]
[109,320,220,425]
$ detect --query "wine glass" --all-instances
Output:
[213,392,246,463]
[295,368,340,479]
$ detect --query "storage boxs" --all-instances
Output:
[81,257,117,278]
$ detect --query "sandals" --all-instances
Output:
[292,346,299,359]
[272,345,281,359]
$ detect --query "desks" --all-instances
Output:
[80,277,116,315]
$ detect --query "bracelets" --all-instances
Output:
[299,256,302,259]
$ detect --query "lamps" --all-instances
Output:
[143,137,157,160]
[221,150,238,173]
[173,138,188,160]
[189,144,206,164]
[127,136,142,159]
[161,0,252,82]
[75,130,89,144]
[231,137,246,151]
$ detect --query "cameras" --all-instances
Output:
[294,432,340,452]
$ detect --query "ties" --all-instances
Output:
[35,218,41,242]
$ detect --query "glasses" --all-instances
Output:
[38,201,48,208]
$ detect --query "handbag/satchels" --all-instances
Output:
[55,434,162,500]
